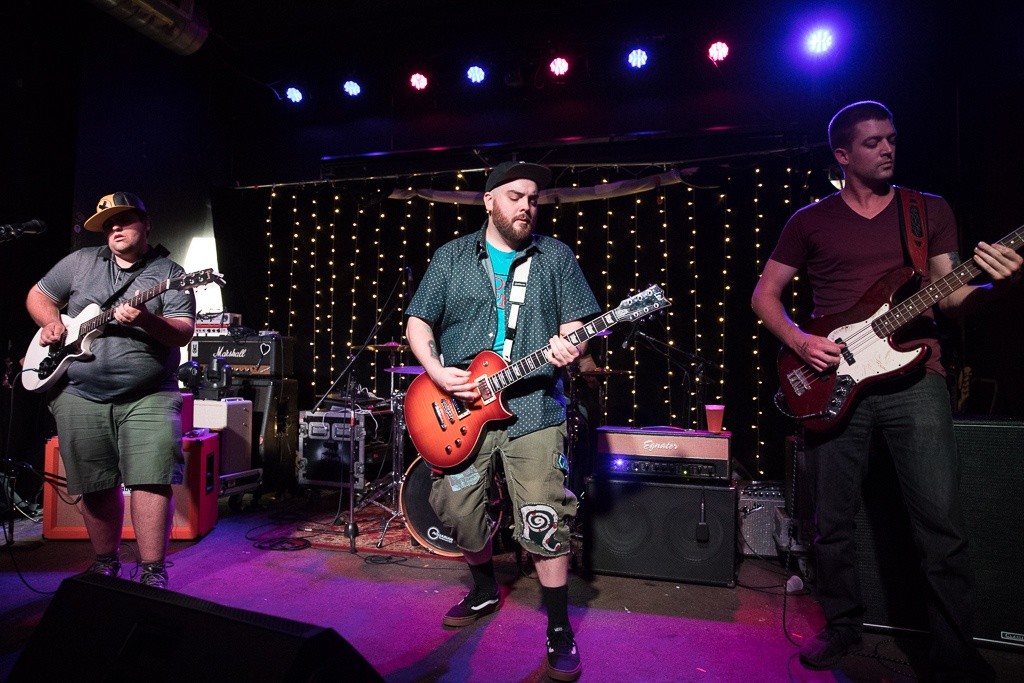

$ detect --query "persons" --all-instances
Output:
[751,100,1024,672]
[406,160,603,683]
[26,191,197,591]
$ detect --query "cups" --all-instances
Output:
[704,404,725,432]
[784,575,803,592]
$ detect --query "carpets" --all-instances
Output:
[255,505,466,562]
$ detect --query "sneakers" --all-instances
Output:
[546,618,581,681]
[442,584,501,626]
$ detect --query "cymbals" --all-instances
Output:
[570,369,636,377]
[383,366,427,375]
[350,341,412,353]
[594,330,612,336]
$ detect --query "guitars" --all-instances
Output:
[404,283,673,469]
[21,267,227,395]
[774,225,1024,432]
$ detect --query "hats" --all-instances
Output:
[485,161,551,191]
[83,192,145,232]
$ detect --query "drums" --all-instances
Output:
[398,454,507,558]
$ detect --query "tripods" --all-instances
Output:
[297,346,406,554]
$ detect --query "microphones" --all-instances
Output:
[407,271,414,299]
[696,495,709,543]
[622,319,639,349]
[0,220,46,235]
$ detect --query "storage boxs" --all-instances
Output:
[298,410,392,490]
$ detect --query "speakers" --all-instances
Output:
[852,420,1024,650]
[8,571,389,683]
[738,483,788,556]
[41,376,300,540]
[580,476,735,587]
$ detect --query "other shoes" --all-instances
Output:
[129,559,174,591]
[799,621,864,667]
[933,651,1000,683]
[83,553,123,579]
